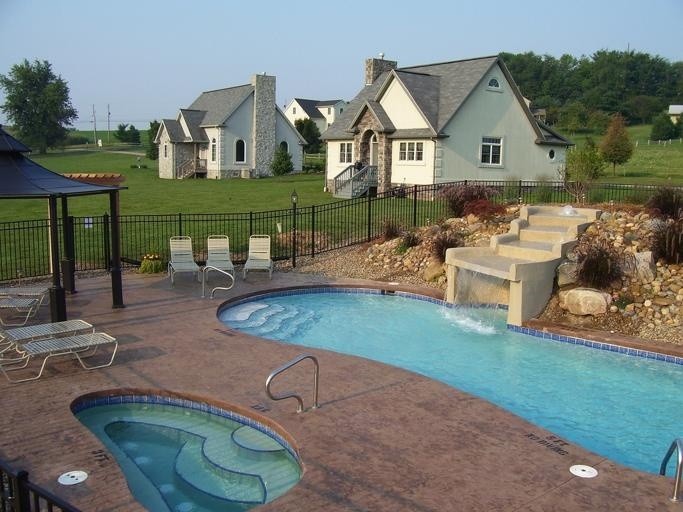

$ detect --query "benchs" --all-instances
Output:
[0,331,121,386]
[1,318,95,365]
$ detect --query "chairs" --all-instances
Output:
[203,233,235,281]
[241,232,275,281]
[165,235,202,286]
